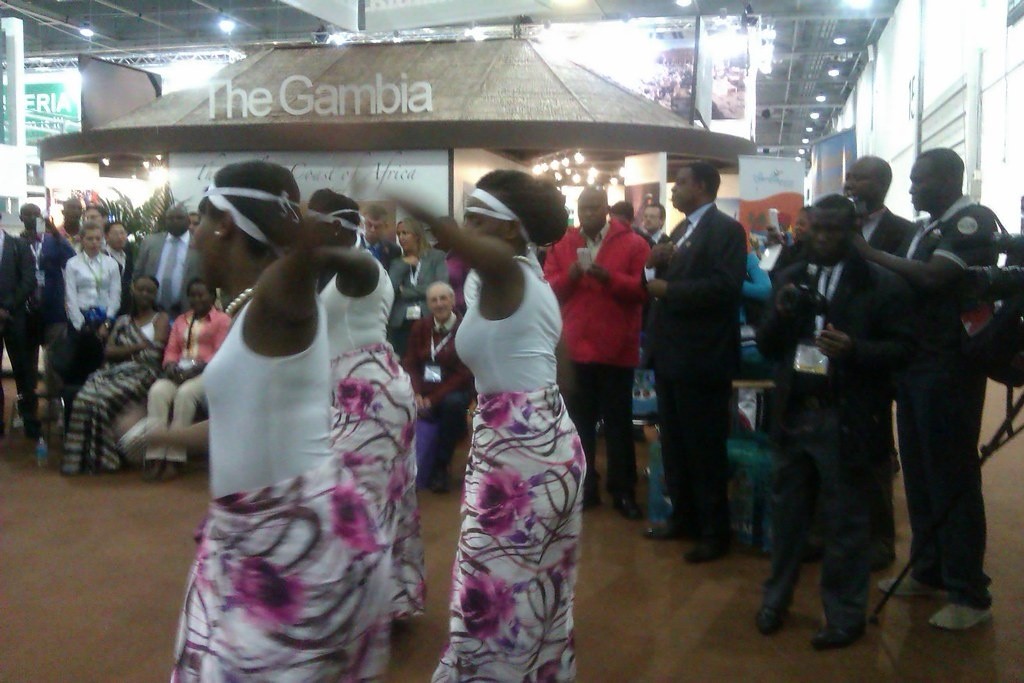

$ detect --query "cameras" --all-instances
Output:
[780,284,827,317]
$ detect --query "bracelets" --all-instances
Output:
[104,321,112,328]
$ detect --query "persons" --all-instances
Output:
[0,203,231,483]
[753,148,999,650]
[360,202,477,492]
[306,185,428,634]
[529,187,670,522]
[380,166,587,682]
[168,160,388,683]
[645,163,746,563]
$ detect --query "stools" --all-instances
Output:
[6,369,70,465]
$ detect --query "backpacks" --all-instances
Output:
[969,205,1024,385]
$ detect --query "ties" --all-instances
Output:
[672,219,690,245]
[161,237,179,310]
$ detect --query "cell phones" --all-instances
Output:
[577,248,593,270]
[768,207,785,244]
[36,218,45,234]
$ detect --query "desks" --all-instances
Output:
[728,375,776,408]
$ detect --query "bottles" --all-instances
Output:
[634,370,641,397]
[643,373,650,398]
[37,438,48,468]
[638,193,652,233]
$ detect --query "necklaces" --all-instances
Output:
[225,288,254,316]
[513,254,529,263]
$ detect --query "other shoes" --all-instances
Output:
[583,492,598,508]
[163,462,180,483]
[929,603,992,630]
[613,490,643,520]
[428,461,447,493]
[879,577,949,596]
[642,524,693,540]
[756,606,784,633]
[684,536,729,561]
[24,419,42,438]
[811,616,865,650]
[143,461,160,482]
